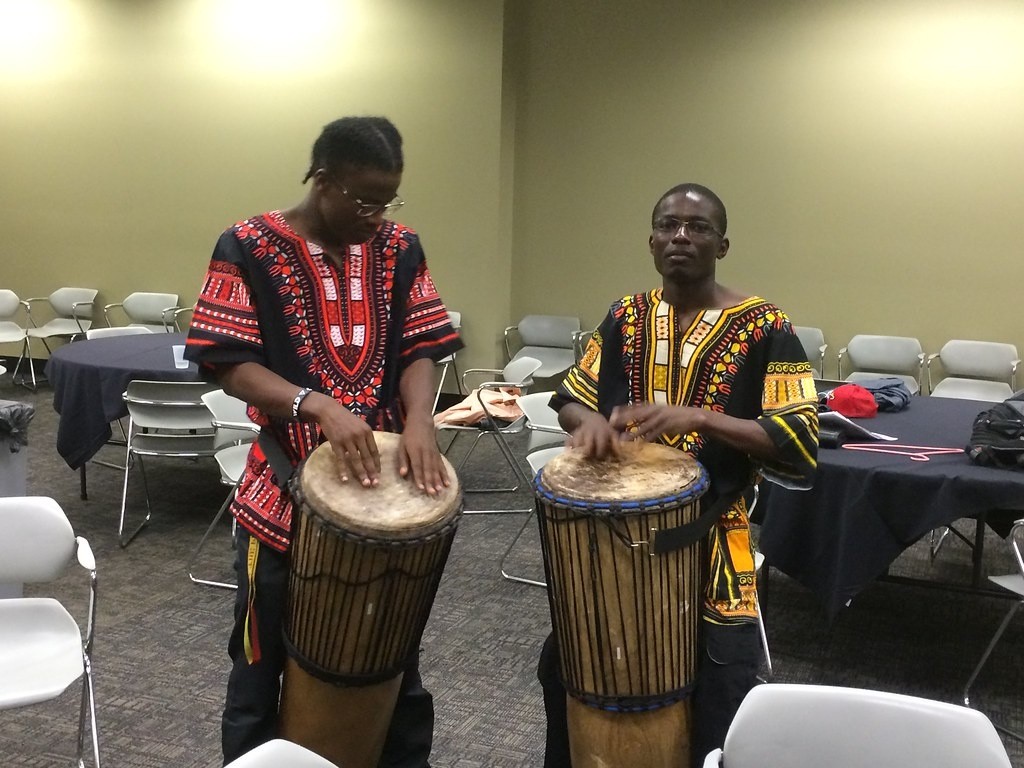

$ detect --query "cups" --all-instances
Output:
[171,344,190,370]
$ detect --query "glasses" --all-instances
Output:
[653,217,723,238]
[324,167,404,217]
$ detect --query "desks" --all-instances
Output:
[43,332,202,501]
[752,396,1024,649]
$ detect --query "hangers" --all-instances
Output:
[842,443,966,462]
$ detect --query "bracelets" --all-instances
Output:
[292,388,313,423]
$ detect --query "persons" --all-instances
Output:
[538,184,819,768]
[182,117,466,768]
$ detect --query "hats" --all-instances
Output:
[818,384,878,418]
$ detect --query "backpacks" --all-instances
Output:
[964,391,1024,470]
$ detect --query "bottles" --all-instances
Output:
[479,417,509,429]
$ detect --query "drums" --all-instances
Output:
[274,432,464,768]
[533,440,711,768]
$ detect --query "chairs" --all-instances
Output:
[223,739,338,768]
[434,313,1024,767]
[0,287,592,589]
[0,495,102,768]
